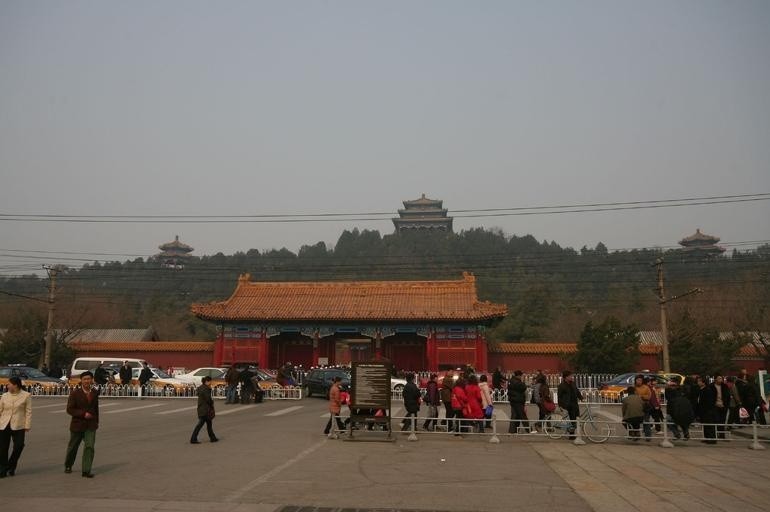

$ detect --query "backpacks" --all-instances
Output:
[423,392,430,405]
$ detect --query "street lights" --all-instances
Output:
[654,256,670,371]
[466,362,470,371]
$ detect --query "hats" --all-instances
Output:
[561,368,575,378]
[511,370,524,377]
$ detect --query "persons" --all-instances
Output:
[0,376,32,479]
[94,364,108,387]
[324,377,388,434]
[138,361,153,400]
[507,369,584,442]
[400,368,493,437]
[492,367,509,401]
[223,361,265,404]
[621,373,769,445]
[119,361,132,387]
[64,370,99,478]
[190,375,219,444]
[40,364,49,377]
[391,365,405,378]
[276,362,296,388]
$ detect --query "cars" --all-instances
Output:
[108,364,196,396]
[599,371,670,404]
[303,368,351,400]
[0,363,66,396]
[208,367,278,398]
[86,366,122,386]
[348,371,408,395]
[418,370,509,400]
[657,369,684,385]
[172,367,230,387]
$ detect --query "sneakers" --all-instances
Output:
[628,433,641,442]
[190,438,202,444]
[529,430,539,434]
[65,464,72,473]
[81,471,95,478]
[701,439,719,445]
[672,433,680,442]
[401,421,495,434]
[656,430,665,436]
[683,432,689,441]
[210,437,220,442]
[0,467,16,478]
[568,432,577,441]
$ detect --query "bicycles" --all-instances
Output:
[540,397,612,443]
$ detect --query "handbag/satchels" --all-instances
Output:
[461,402,471,418]
[739,406,750,418]
[416,398,421,409]
[642,400,655,415]
[485,405,493,417]
[543,399,556,412]
[207,400,216,420]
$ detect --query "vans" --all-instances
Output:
[69,358,152,393]
